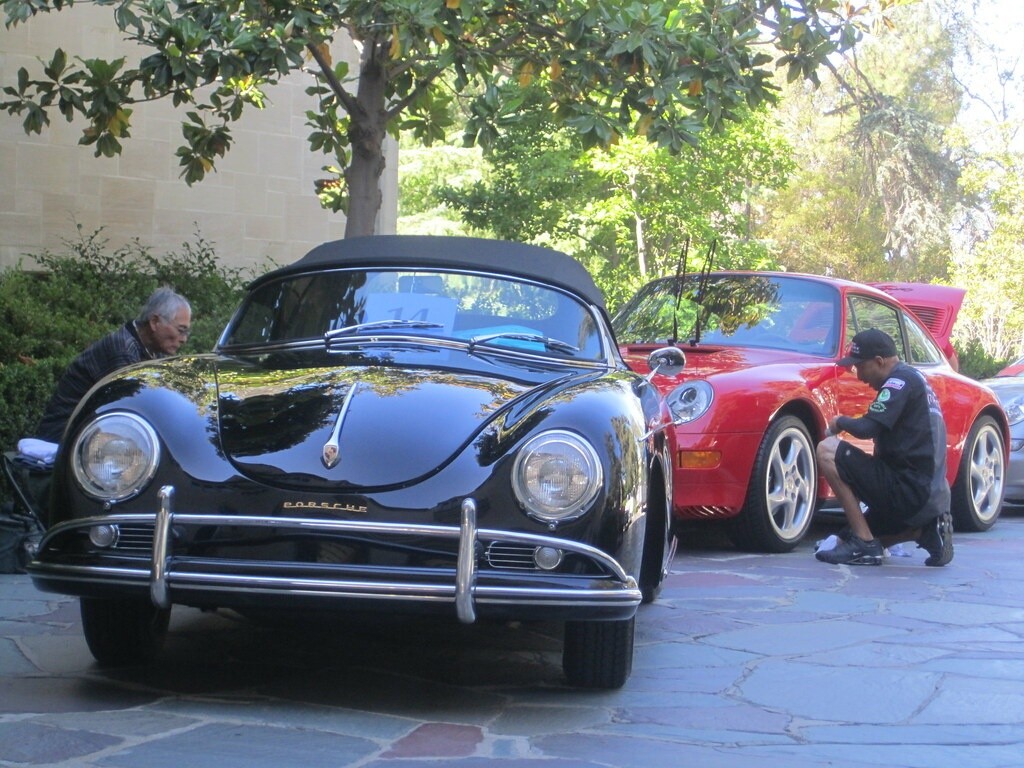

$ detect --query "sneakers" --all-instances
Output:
[816,536,883,565]
[916,512,954,566]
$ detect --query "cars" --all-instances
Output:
[976,355,1024,512]
[603,269,1011,554]
[22,233,688,693]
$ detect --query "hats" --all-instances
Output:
[835,326,898,367]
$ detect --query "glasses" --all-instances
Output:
[159,313,194,337]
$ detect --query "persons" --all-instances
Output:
[36,287,192,529]
[814,329,954,566]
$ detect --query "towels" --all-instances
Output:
[18,438,59,464]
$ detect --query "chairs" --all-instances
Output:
[2,450,53,535]
